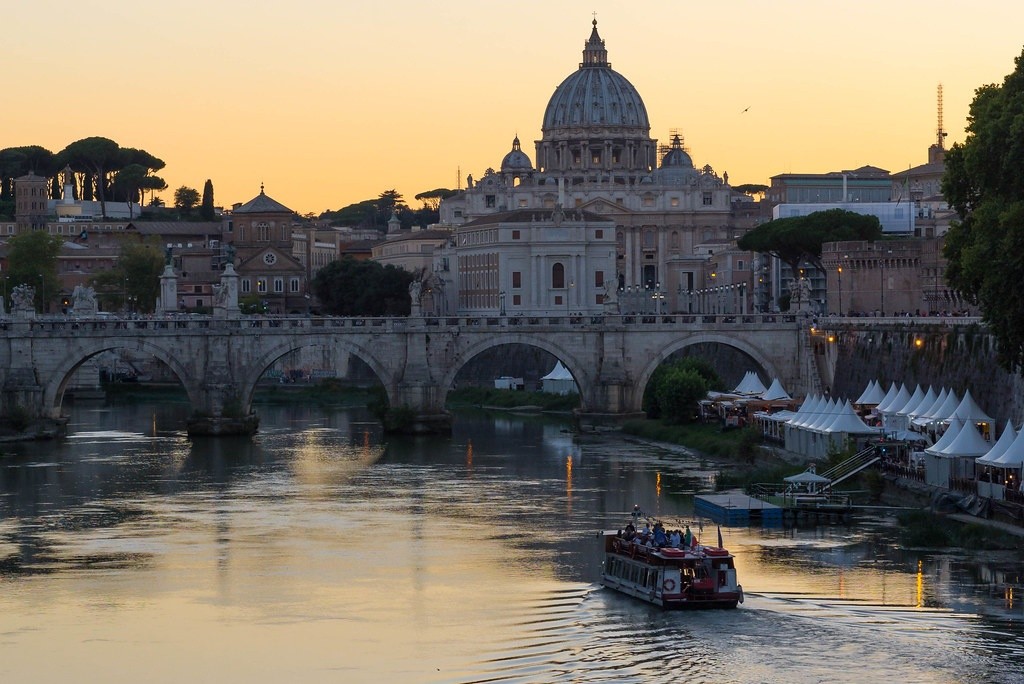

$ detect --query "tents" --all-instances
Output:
[875,382,899,414]
[920,387,960,426]
[912,386,947,426]
[758,378,790,400]
[993,424,1024,488]
[974,419,1018,482]
[783,472,832,505]
[784,392,875,458]
[855,380,874,404]
[733,370,767,393]
[938,415,992,484]
[943,389,995,441]
[896,384,925,429]
[862,380,886,404]
[881,383,912,430]
[923,413,964,490]
[539,359,580,394]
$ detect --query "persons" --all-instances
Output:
[621,522,687,550]
[883,456,926,481]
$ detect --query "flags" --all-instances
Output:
[685,528,698,547]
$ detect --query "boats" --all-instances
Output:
[596,504,738,609]
[767,463,850,504]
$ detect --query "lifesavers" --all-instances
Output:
[664,578,675,591]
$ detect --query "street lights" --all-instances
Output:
[39,269,45,314]
[4,276,10,311]
[690,281,747,314]
[636,284,640,310]
[627,285,630,310]
[620,287,624,311]
[656,282,660,314]
[644,284,649,312]
[500,290,506,316]
[567,279,574,316]
[123,275,129,305]
